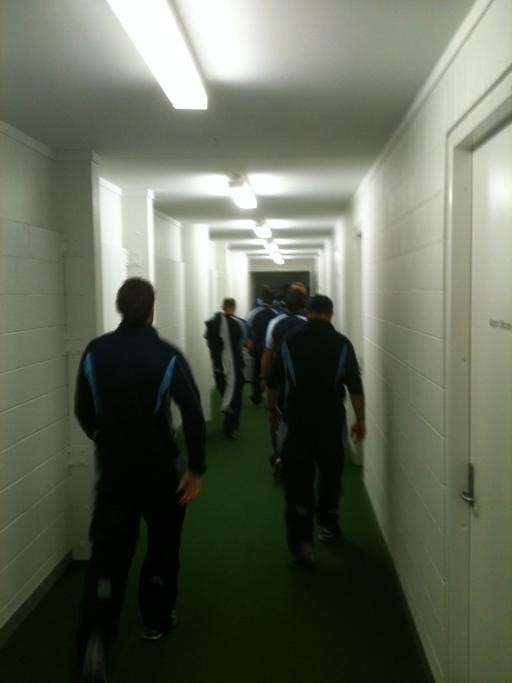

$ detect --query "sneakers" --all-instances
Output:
[318,518,342,541]
[140,609,177,640]
[290,546,316,566]
[272,458,286,487]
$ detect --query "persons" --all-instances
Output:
[71,278,208,682]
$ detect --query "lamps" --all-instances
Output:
[101,1,211,112]
[226,171,288,267]
